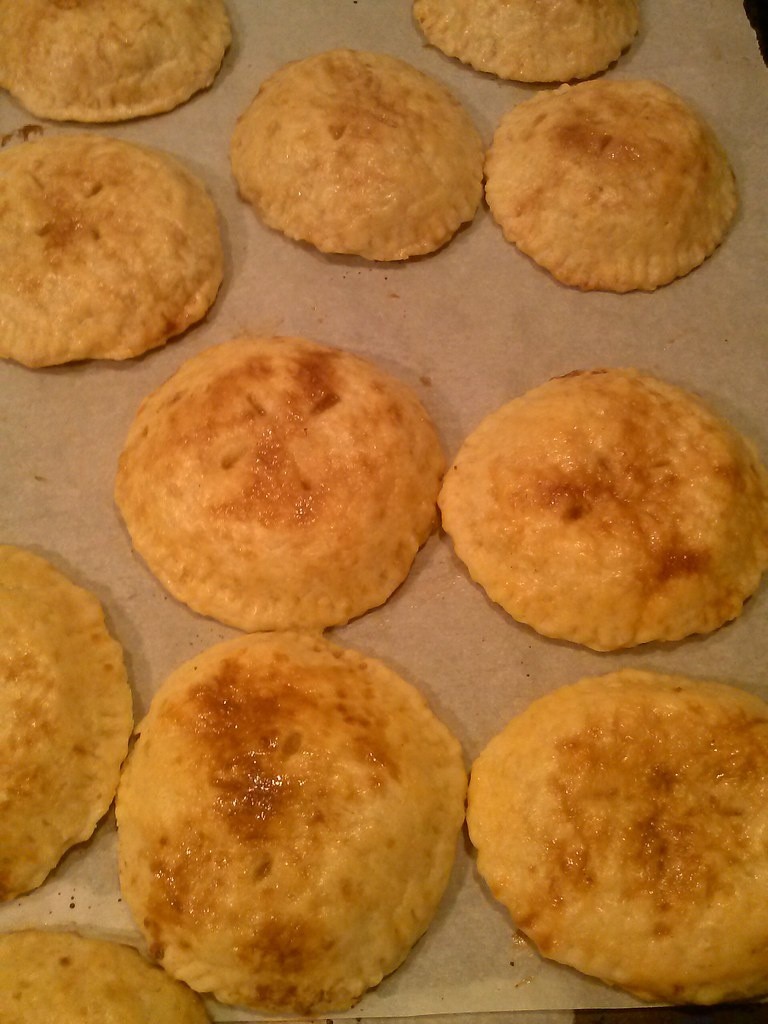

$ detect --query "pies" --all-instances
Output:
[0,0,768,1024]
